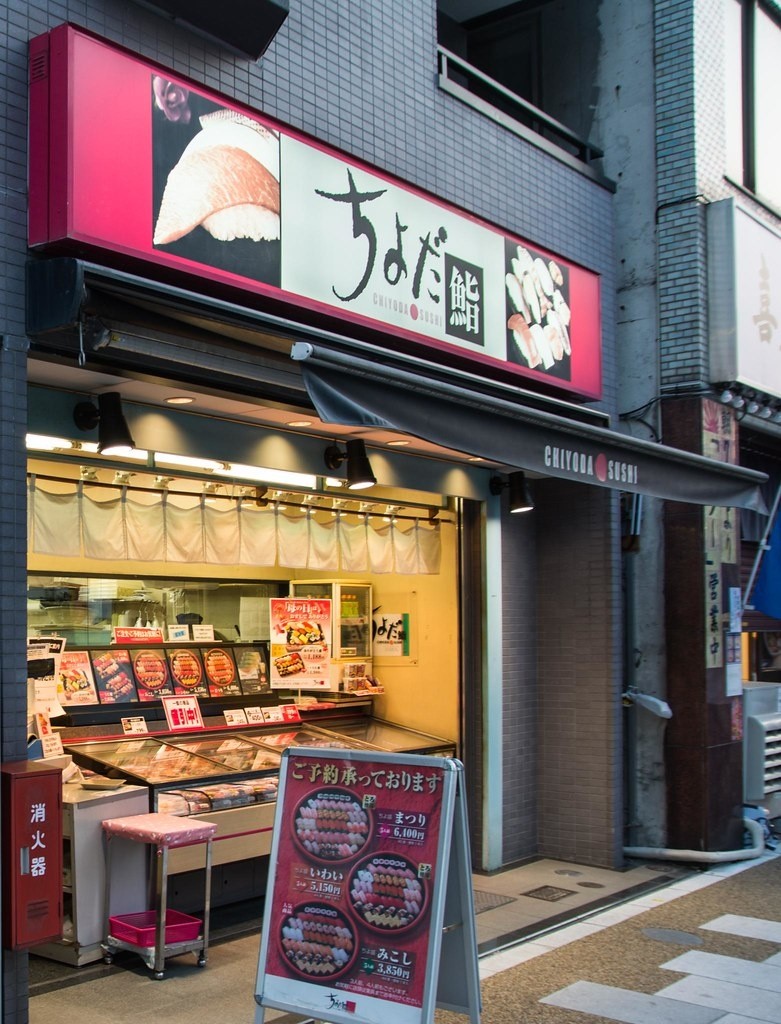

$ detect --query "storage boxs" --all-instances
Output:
[0,761,64,951]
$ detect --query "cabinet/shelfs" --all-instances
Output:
[30,781,151,969]
[101,812,217,979]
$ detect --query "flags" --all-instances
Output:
[754,501,781,618]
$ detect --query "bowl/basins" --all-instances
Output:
[291,786,374,866]
[134,650,167,690]
[40,587,80,606]
[46,606,90,625]
[277,898,361,979]
[346,851,429,936]
[171,649,202,689]
[204,649,234,687]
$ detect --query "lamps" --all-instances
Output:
[154,477,173,489]
[300,495,318,513]
[113,471,131,486]
[74,392,135,456]
[489,471,535,514]
[325,439,377,490]
[384,505,401,522]
[331,499,348,516]
[359,502,373,519]
[270,492,288,510]
[80,465,99,489]
[200,483,216,501]
[237,487,253,506]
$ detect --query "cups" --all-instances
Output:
[344,663,368,691]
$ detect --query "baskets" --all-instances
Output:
[109,908,202,947]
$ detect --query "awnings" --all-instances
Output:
[85,276,769,514]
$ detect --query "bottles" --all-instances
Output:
[112,611,158,626]
[306,595,358,618]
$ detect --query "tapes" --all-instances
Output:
[27,732,38,743]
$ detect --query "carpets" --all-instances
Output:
[475,889,518,913]
